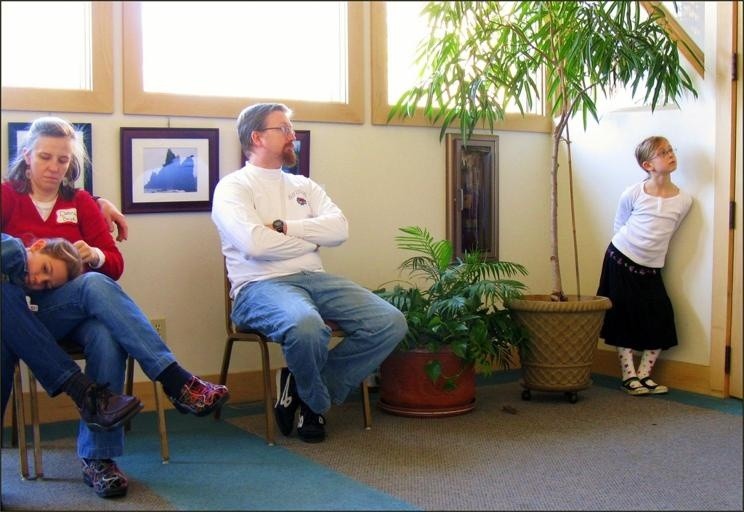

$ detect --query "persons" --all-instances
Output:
[0,196,145,433]
[0,232,85,294]
[596,136,695,398]
[0,115,230,499]
[209,101,409,445]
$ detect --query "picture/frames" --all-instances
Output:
[2,117,95,198]
[117,124,220,217]
[240,127,312,182]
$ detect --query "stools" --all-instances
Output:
[8,319,170,487]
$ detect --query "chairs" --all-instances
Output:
[210,247,375,447]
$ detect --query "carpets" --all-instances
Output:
[219,376,742,511]
[1,433,182,511]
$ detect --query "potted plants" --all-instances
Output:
[383,1,702,393]
[372,223,531,415]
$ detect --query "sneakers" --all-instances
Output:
[641,376,668,394]
[619,377,649,396]
[164,375,232,418]
[81,457,130,499]
[273,366,296,436]
[297,403,326,443]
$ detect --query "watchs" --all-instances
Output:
[273,220,284,233]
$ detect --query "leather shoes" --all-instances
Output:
[78,381,144,433]
